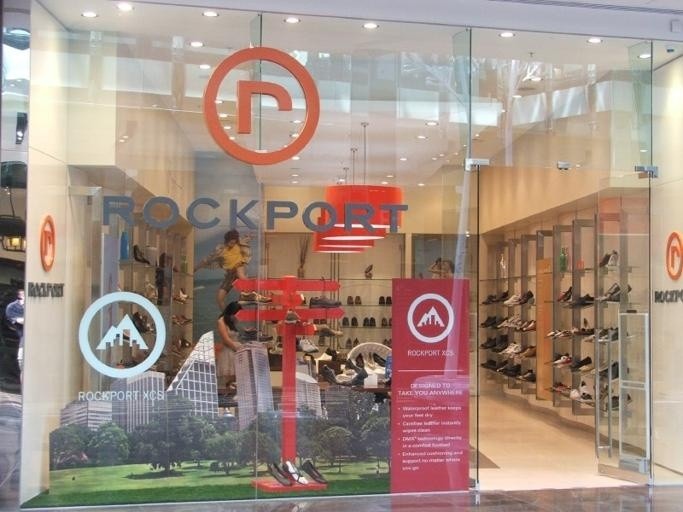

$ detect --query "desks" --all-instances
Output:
[316,374,393,400]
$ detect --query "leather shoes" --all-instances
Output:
[267,461,291,486]
[303,459,327,484]
[283,460,308,484]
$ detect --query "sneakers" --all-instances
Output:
[480,251,633,411]
[238,292,392,386]
[128,245,192,349]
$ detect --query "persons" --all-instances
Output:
[3,288,25,393]
[215,302,243,397]
[193,229,252,308]
[427,257,454,279]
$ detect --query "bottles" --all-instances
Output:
[559,247,568,271]
[498,254,507,278]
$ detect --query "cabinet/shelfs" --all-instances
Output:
[259,227,413,358]
[479,225,640,420]
[113,210,194,384]
